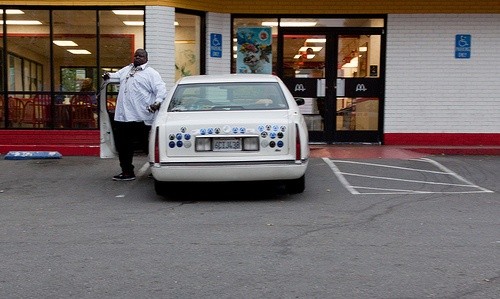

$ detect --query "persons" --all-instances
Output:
[102,49,167,181]
[77,78,98,104]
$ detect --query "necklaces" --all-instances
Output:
[124,66,138,93]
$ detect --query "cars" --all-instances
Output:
[97,73,310,198]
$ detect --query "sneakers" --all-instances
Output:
[112,172,135,181]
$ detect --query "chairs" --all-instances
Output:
[0,95,113,129]
[183,88,198,105]
